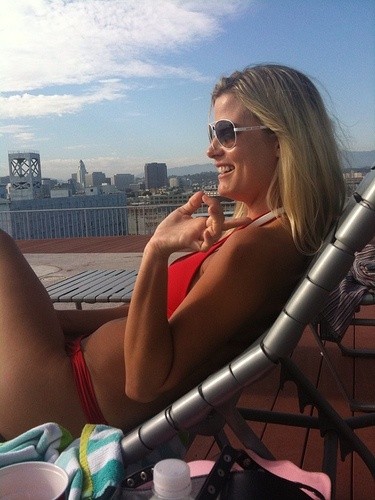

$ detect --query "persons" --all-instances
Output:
[0,64,344,444]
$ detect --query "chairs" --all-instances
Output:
[46,269,375,358]
[119,166,375,500]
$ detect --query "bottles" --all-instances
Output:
[149,458,194,500]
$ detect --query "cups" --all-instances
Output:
[0,461,69,500]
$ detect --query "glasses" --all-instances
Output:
[208,119,268,149]
